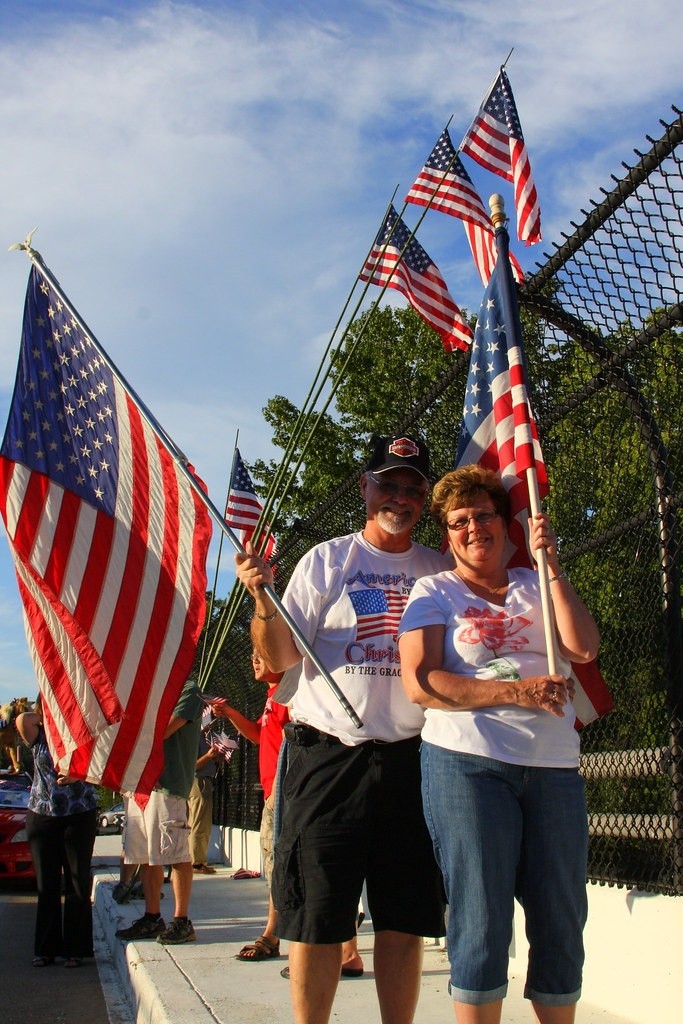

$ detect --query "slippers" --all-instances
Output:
[342,968,364,977]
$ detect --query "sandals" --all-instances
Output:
[240,936,280,961]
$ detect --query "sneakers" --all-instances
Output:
[156,919,197,944]
[115,917,166,940]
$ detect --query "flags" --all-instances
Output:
[0,264,213,813]
[228,448,278,575]
[199,693,234,761]
[407,128,526,289]
[361,204,474,352]
[464,65,542,247]
[441,227,550,570]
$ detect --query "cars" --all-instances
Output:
[98,802,125,828]
[0,768,35,884]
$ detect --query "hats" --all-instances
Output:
[368,434,430,483]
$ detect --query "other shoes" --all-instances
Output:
[193,864,216,874]
[112,882,130,903]
[131,885,163,899]
[64,957,83,967]
[280,966,290,980]
[32,957,50,967]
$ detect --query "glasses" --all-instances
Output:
[369,475,429,498]
[250,653,264,661]
[444,508,500,531]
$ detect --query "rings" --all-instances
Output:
[552,692,556,701]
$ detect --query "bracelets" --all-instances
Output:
[549,573,564,583]
[254,609,278,622]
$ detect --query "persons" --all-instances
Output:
[187,729,218,874]
[235,435,457,1024]
[397,464,601,1024]
[212,645,365,978]
[112,817,165,905]
[116,680,196,945]
[16,693,99,968]
